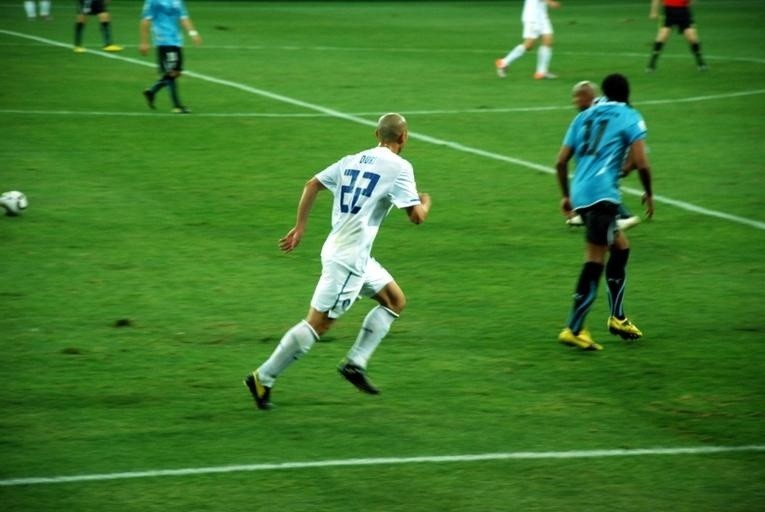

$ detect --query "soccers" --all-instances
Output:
[1,191,27,217]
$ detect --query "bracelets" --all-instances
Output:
[188,30,197,37]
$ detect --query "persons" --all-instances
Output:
[496,1,562,83]
[71,1,125,54]
[561,79,644,233]
[241,113,432,413]
[554,71,656,352]
[23,0,53,24]
[645,0,710,76]
[137,0,200,114]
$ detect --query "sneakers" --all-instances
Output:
[336,356,380,394]
[614,215,641,230]
[243,371,275,410]
[144,90,191,113]
[559,327,604,350]
[495,60,558,80]
[566,214,584,225]
[607,316,643,339]
